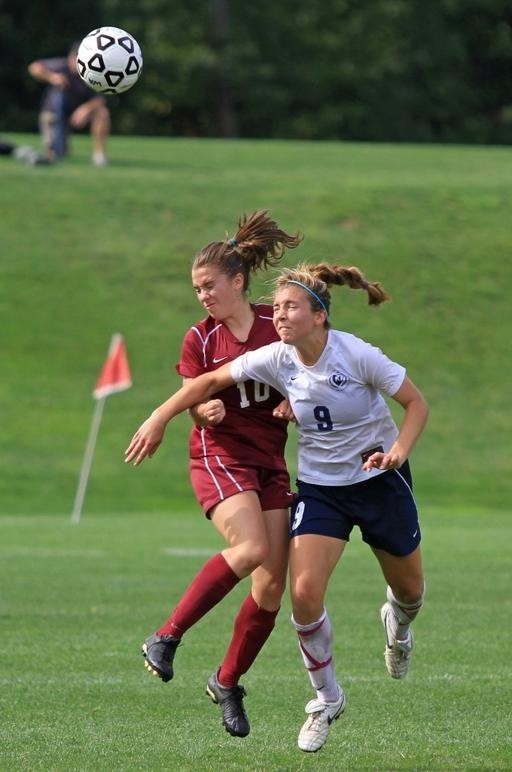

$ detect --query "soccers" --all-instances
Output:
[76,26,144,95]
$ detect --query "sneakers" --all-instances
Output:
[297,684,345,752]
[381,603,412,678]
[142,631,181,682]
[206,666,250,737]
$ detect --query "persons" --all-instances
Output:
[25,40,114,170]
[121,258,433,753]
[0,141,59,169]
[138,209,302,740]
